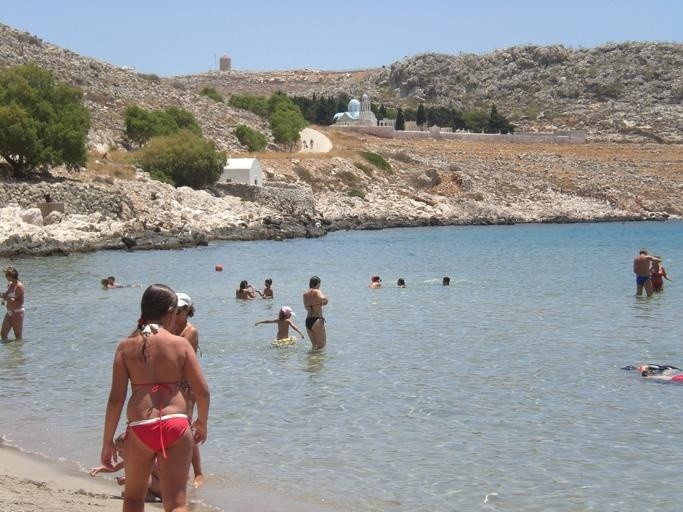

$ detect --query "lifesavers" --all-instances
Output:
[271,336,296,348]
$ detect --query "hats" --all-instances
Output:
[281,306,295,316]
[174,292,192,308]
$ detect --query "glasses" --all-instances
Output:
[175,309,181,316]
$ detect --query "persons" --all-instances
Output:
[171,292,204,487]
[369,275,381,288]
[649,256,671,292]
[633,248,661,298]
[100,279,110,288]
[443,277,450,284]
[100,283,210,511]
[397,278,405,288]
[622,362,683,384]
[107,276,117,288]
[255,279,273,299]
[255,306,304,340]
[236,280,256,299]
[0,267,25,341]
[90,432,161,503]
[303,276,328,349]
